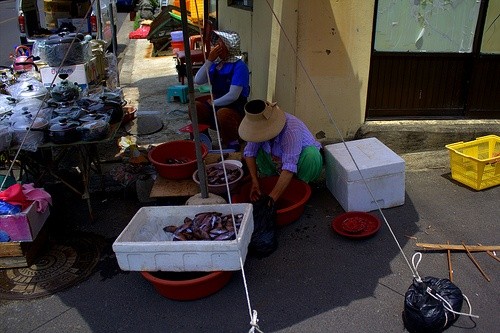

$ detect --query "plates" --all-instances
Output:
[219,159,243,168]
[331,211,381,237]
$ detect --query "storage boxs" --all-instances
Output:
[324,137,406,213]
[41,54,104,93]
[445,135,500,190]
[42,0,91,33]
[0,199,50,242]
[112,202,253,272]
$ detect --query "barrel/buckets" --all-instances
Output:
[171,41,184,51]
[170,31,183,41]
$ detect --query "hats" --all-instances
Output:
[210,29,243,64]
[238,99,286,142]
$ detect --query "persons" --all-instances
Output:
[188,30,249,143]
[238,99,323,205]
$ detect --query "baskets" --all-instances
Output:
[444,135,500,191]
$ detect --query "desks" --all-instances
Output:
[0,86,125,222]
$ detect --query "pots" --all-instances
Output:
[45,27,91,68]
[0,68,127,148]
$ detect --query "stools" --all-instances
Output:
[166,84,189,104]
[176,50,204,85]
[189,35,203,50]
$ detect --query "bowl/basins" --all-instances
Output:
[193,163,244,194]
[231,176,311,225]
[138,268,234,300]
[148,139,208,180]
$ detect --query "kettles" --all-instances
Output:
[9,46,34,74]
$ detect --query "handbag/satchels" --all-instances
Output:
[248,195,279,254]
